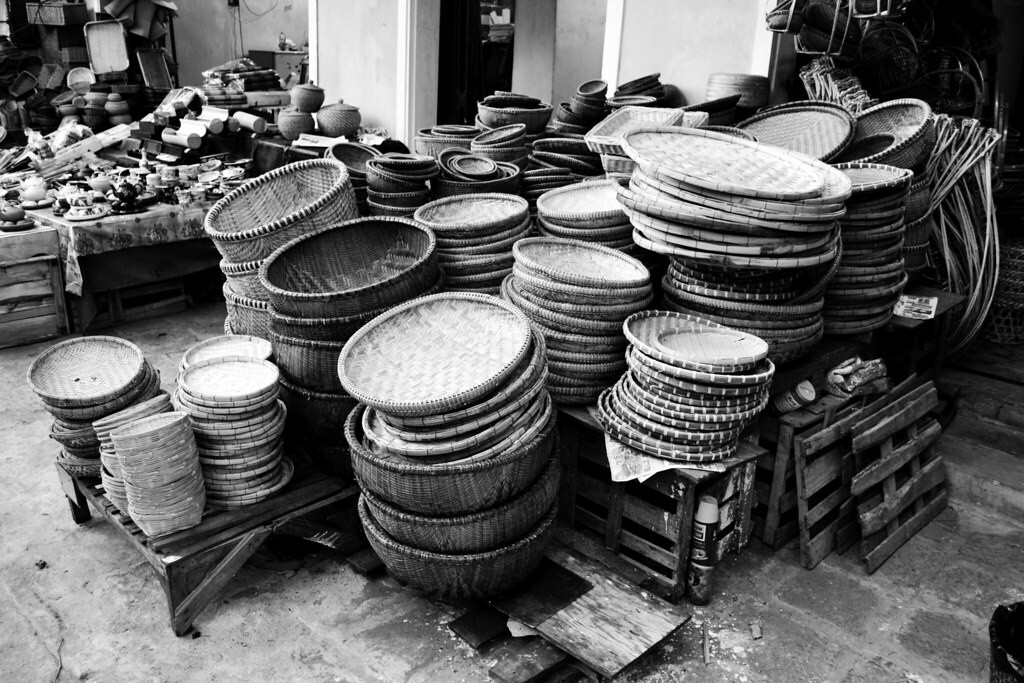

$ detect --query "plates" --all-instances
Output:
[0,189,156,231]
[198,159,255,196]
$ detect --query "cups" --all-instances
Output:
[146,163,207,205]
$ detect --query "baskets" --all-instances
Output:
[27,0,1024,606]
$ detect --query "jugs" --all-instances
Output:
[0,166,160,222]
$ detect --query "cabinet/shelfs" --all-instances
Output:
[248,50,308,80]
